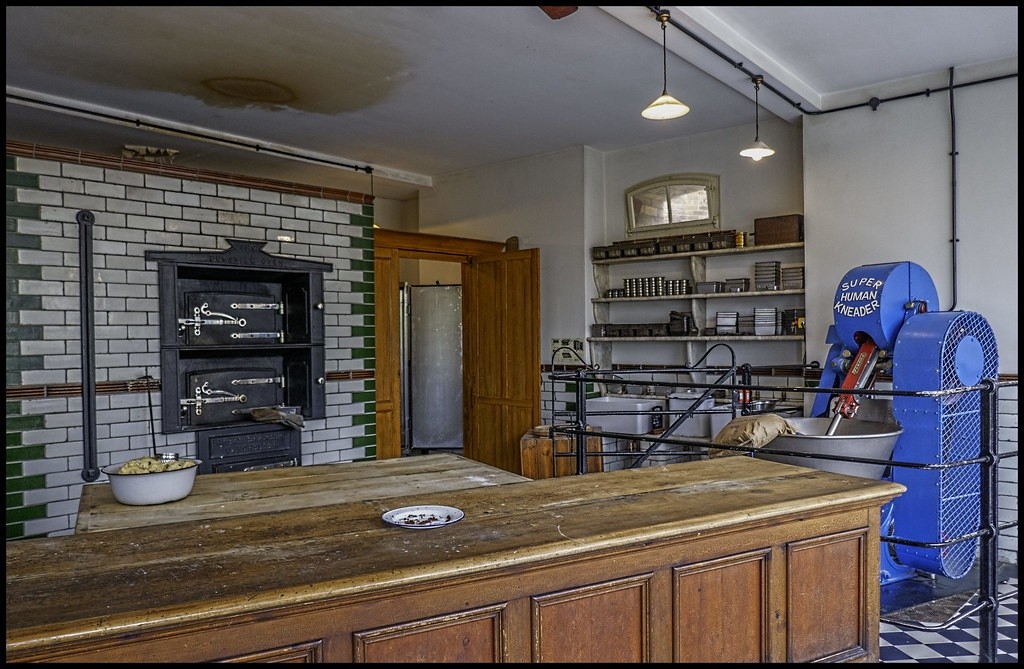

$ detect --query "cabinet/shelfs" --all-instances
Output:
[144,239,335,435]
[586,242,805,444]
[6,454,908,663]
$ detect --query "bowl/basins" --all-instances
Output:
[101,460,202,505]
[730,399,779,409]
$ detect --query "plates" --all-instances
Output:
[382,506,465,529]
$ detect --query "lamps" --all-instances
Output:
[640,9,690,120]
[739,75,775,161]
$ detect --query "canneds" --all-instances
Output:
[735,232,747,248]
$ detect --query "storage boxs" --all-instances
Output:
[754,214,804,246]
[710,404,802,440]
[585,396,667,435]
